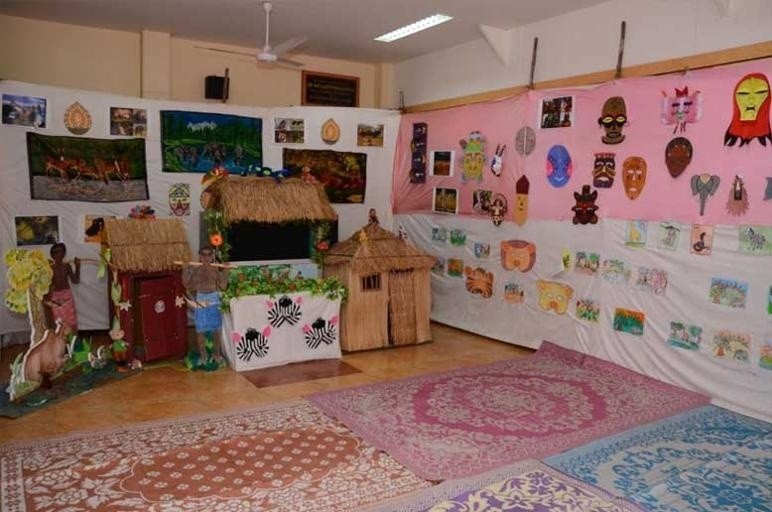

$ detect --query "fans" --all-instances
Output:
[192,0,310,70]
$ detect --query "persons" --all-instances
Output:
[44,241,81,336]
[182,247,230,362]
